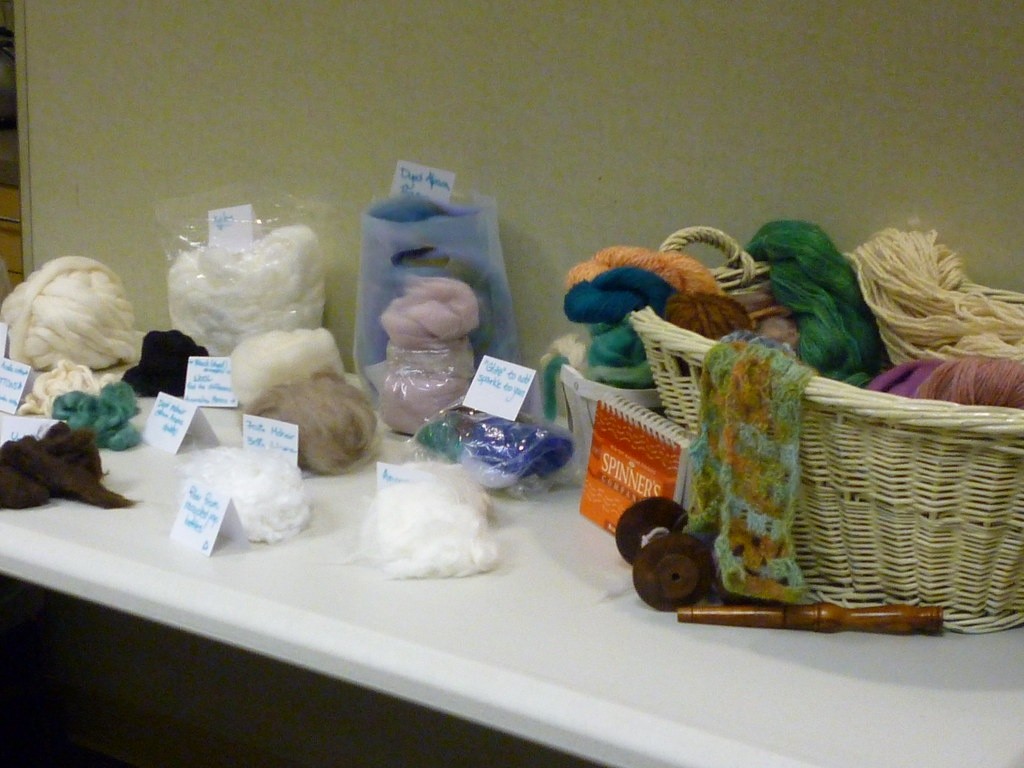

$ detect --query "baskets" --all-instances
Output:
[623,222,1024,636]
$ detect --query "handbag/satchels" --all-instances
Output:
[354,192,545,420]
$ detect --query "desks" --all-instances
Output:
[0,362,1024,768]
